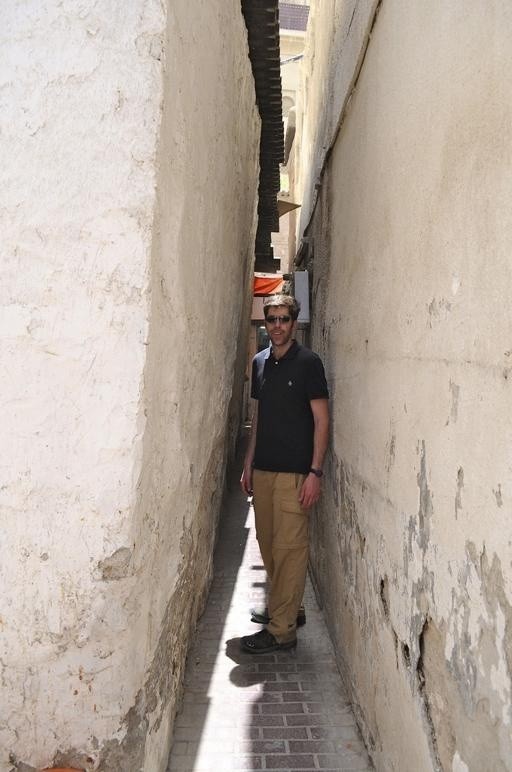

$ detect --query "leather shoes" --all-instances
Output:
[241,629,296,653]
[252,606,306,625]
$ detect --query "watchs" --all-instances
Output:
[310,468,324,477]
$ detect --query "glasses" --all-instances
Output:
[266,315,289,323]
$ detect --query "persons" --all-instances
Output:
[240,295,330,654]
